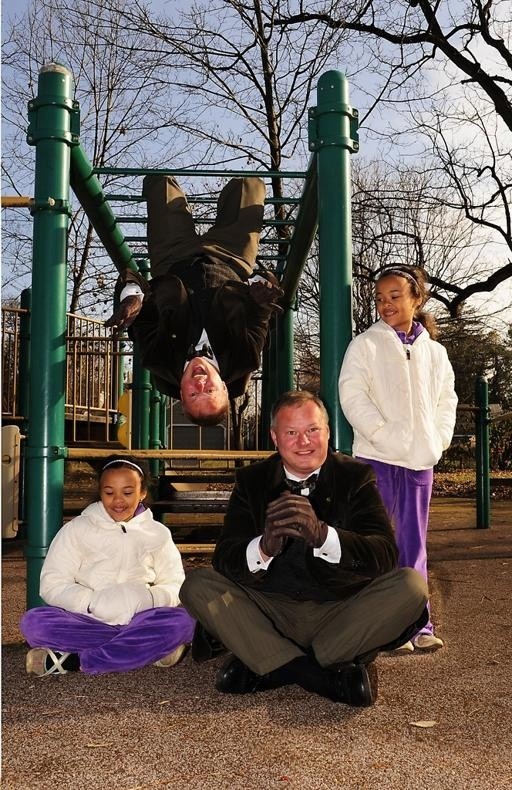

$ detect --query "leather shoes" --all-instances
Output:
[217,655,273,693]
[327,663,372,707]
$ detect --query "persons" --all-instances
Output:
[178,392,429,708]
[20,453,197,679]
[103,173,285,427]
[338,265,459,652]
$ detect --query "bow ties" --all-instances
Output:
[282,474,317,493]
[186,343,213,360]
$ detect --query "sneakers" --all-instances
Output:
[152,643,187,667]
[414,633,444,648]
[25,648,72,676]
[394,641,414,654]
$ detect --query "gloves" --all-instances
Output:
[104,295,142,340]
[260,495,328,557]
[250,271,284,312]
